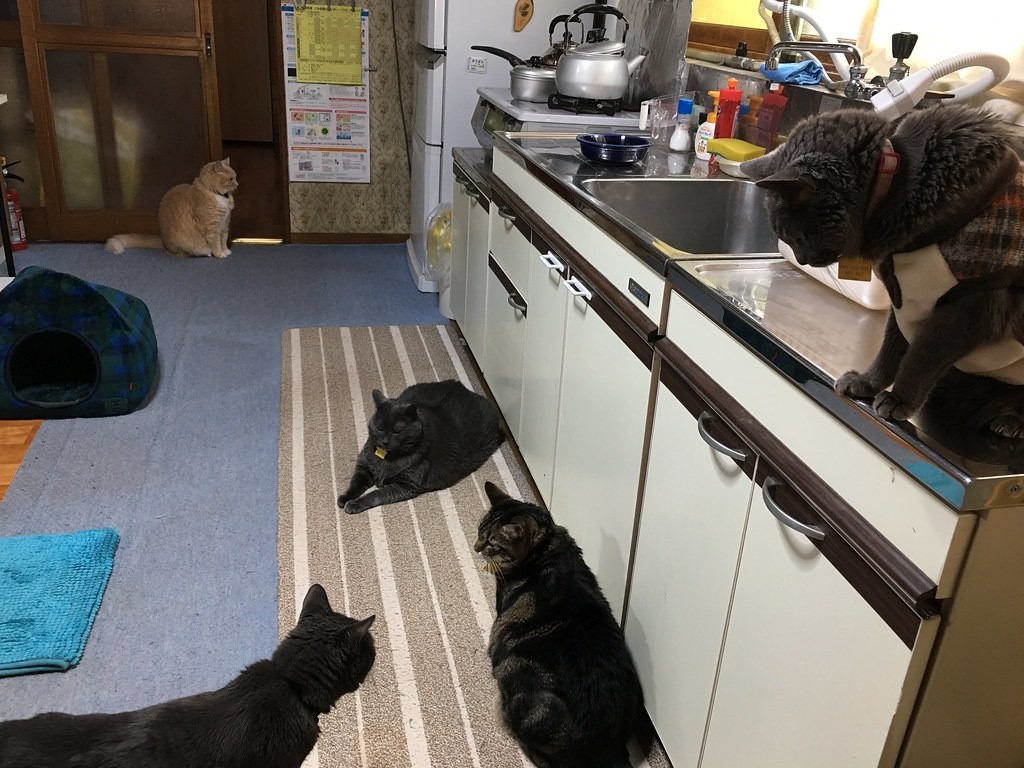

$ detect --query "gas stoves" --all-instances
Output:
[470,85,652,150]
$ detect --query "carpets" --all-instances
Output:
[0,526,121,677]
[0,419,43,506]
[277,323,651,767]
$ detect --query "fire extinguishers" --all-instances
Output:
[0,156,27,251]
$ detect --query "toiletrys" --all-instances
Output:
[694,90,720,162]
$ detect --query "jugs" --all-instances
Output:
[639,91,697,145]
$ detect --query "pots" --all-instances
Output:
[471,46,557,102]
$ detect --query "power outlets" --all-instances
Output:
[466,57,489,74]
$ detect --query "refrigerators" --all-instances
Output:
[406,0,620,292]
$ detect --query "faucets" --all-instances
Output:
[764,39,868,76]
[581,175,782,255]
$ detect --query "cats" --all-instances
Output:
[471,479,652,768]
[0,582,375,767]
[103,157,239,259]
[738,102,1024,472]
[337,379,511,515]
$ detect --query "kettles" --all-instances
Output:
[556,4,647,100]
[540,14,583,69]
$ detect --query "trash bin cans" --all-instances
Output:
[427,202,458,321]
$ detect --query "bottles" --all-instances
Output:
[670,99,693,152]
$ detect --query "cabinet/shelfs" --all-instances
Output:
[622,351,1024,768]
[449,164,659,633]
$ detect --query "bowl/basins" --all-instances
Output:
[576,133,653,163]
